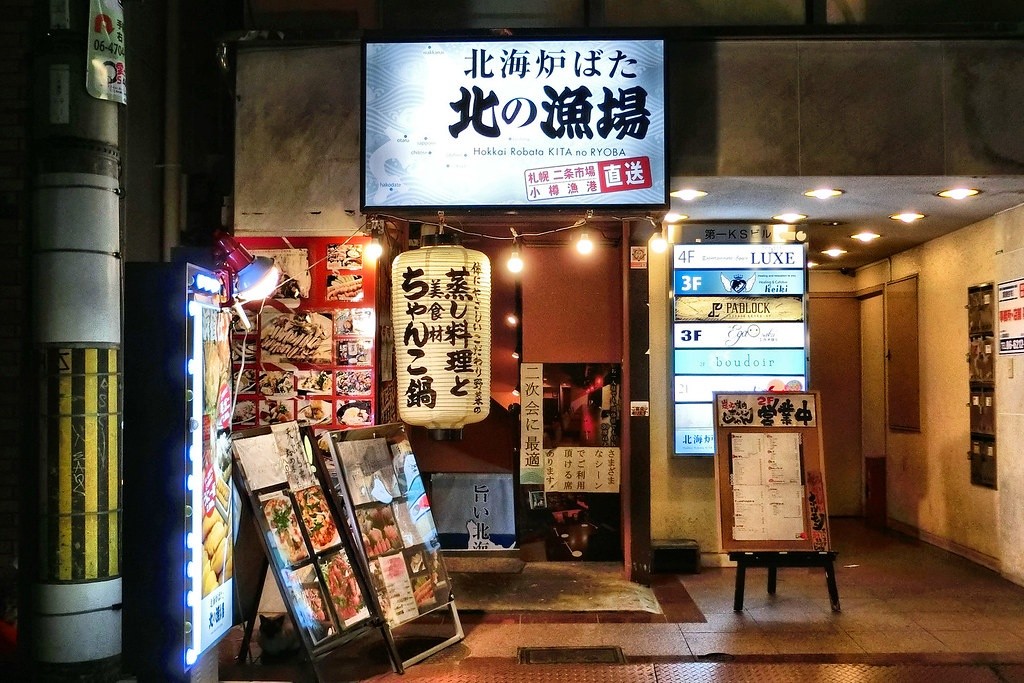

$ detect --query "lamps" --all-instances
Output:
[210,228,280,301]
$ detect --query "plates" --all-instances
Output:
[336,402,370,425]
[297,403,331,427]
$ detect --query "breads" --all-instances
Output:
[203,506,232,597]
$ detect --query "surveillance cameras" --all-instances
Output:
[840,267,850,274]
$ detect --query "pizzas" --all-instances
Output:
[296,486,340,550]
[264,496,308,564]
